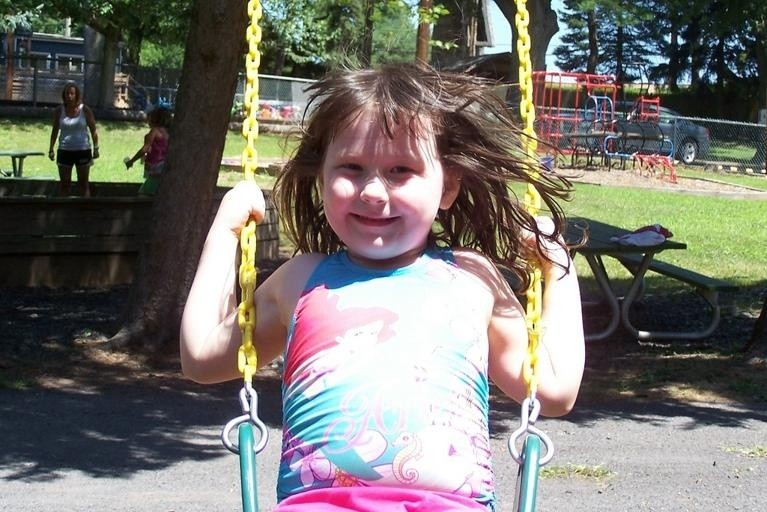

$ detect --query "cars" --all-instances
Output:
[563,101,710,166]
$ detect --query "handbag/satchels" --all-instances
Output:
[618,231,666,247]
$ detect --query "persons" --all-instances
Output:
[180,59,587,510]
[49,83,100,198]
[125,107,174,194]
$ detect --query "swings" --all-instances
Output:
[222,0,544,512]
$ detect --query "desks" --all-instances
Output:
[0,151,45,176]
[551,217,722,344]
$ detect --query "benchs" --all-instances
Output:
[604,252,740,341]
